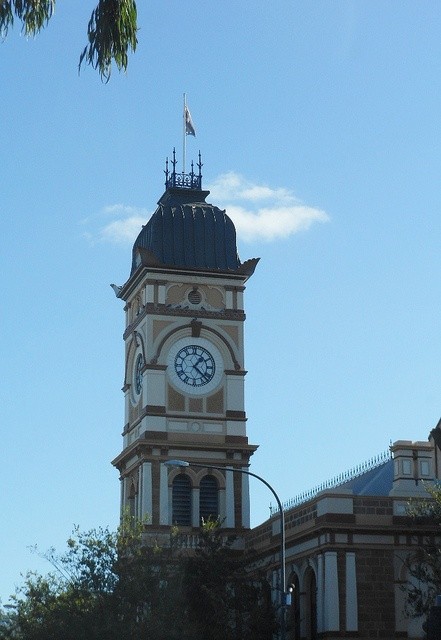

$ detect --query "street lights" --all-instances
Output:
[162,458,297,639]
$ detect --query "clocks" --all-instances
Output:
[134,354,142,395]
[165,335,226,399]
